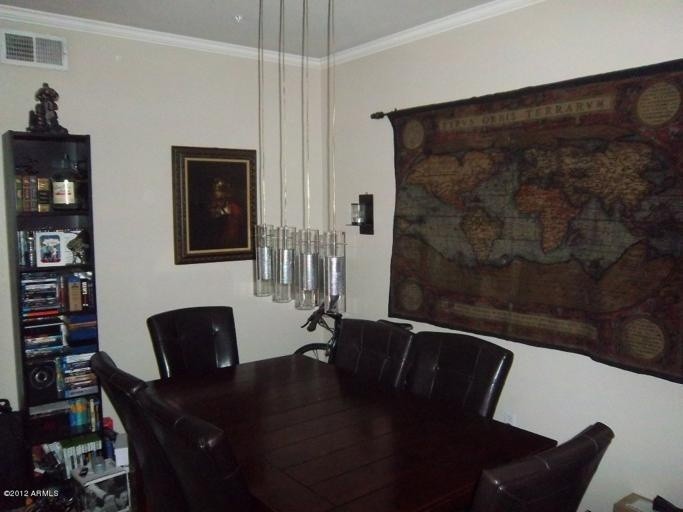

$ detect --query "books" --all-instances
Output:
[15,175,127,487]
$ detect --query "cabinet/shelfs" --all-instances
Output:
[0,130,108,440]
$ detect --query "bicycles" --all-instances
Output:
[292,301,415,364]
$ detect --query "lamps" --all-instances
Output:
[249,1,348,318]
[346,191,378,236]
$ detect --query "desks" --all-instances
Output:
[133,349,559,512]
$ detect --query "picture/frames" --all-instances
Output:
[168,145,256,267]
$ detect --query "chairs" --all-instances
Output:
[472,421,616,512]
[327,315,412,390]
[393,329,514,424]
[146,304,241,379]
[88,345,147,442]
[137,389,252,510]
